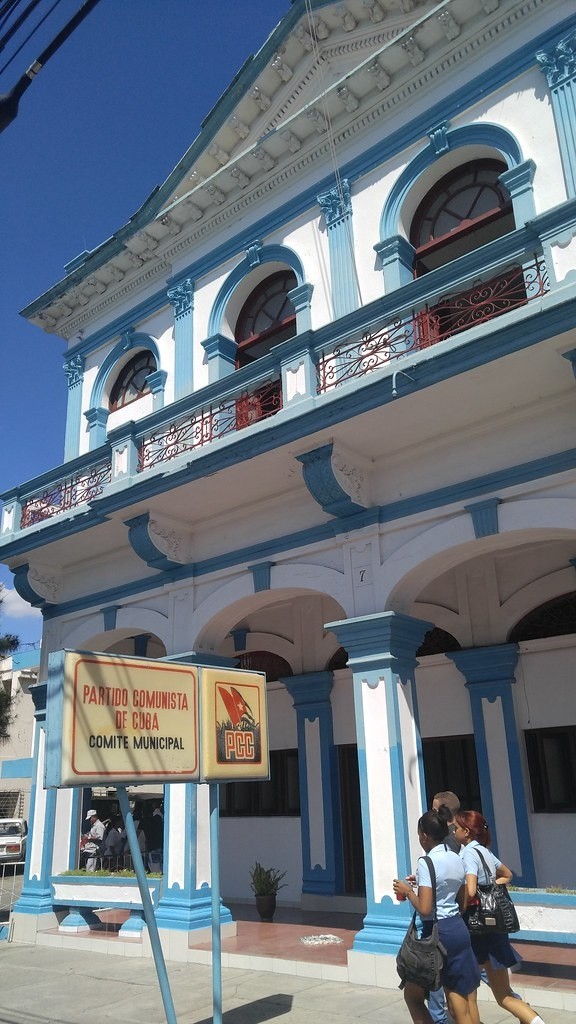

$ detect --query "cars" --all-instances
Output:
[0,818,28,875]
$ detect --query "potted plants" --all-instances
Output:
[248,860,288,918]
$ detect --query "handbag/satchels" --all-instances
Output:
[396,920,447,992]
[456,847,520,935]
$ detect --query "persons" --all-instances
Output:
[392,804,479,1024]
[427,792,529,1024]
[80,798,164,875]
[452,811,545,1024]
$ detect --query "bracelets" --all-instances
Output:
[405,890,415,900]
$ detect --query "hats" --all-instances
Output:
[85,809,97,820]
